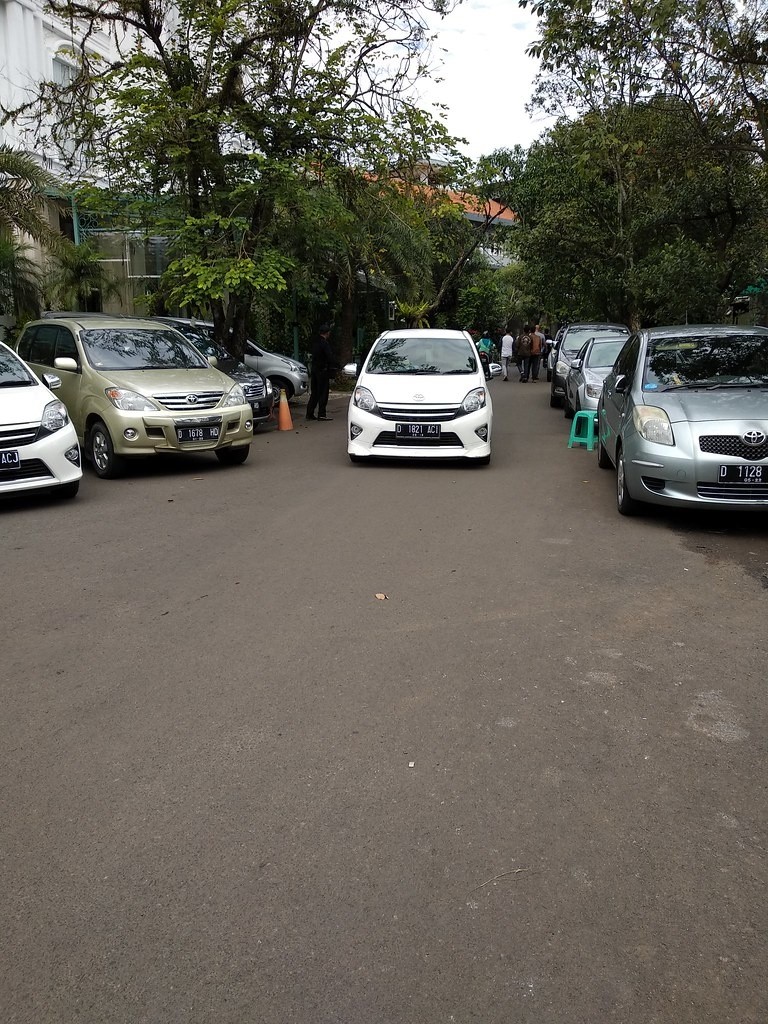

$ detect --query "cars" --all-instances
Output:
[166,317,309,405]
[43,311,274,431]
[598,324,768,513]
[14,317,253,478]
[0,339,84,501]
[341,329,493,465]
[564,336,630,436]
[545,328,564,382]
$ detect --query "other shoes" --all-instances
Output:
[502,377,508,381]
[518,373,537,384]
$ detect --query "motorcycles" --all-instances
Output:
[475,342,495,381]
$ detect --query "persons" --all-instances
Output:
[477,326,550,383]
[305,325,333,422]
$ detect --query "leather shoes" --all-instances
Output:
[306,414,334,421]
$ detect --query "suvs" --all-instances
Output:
[549,321,632,407]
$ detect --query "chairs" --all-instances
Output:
[649,350,693,389]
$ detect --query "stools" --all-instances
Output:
[566,409,601,451]
